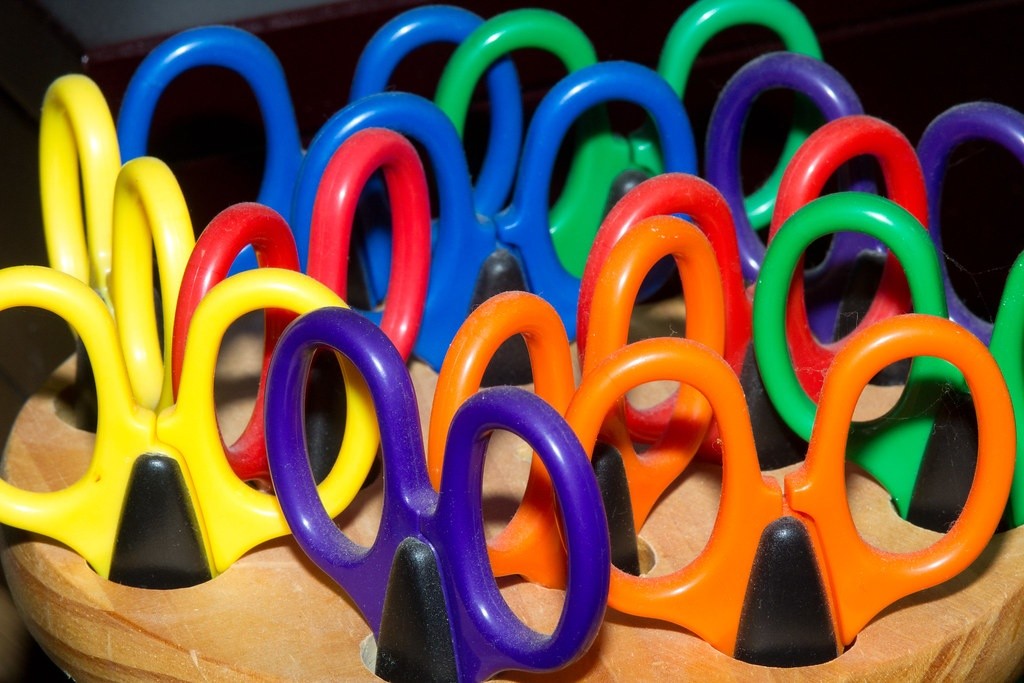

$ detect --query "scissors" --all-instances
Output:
[0,0,1024,683]
